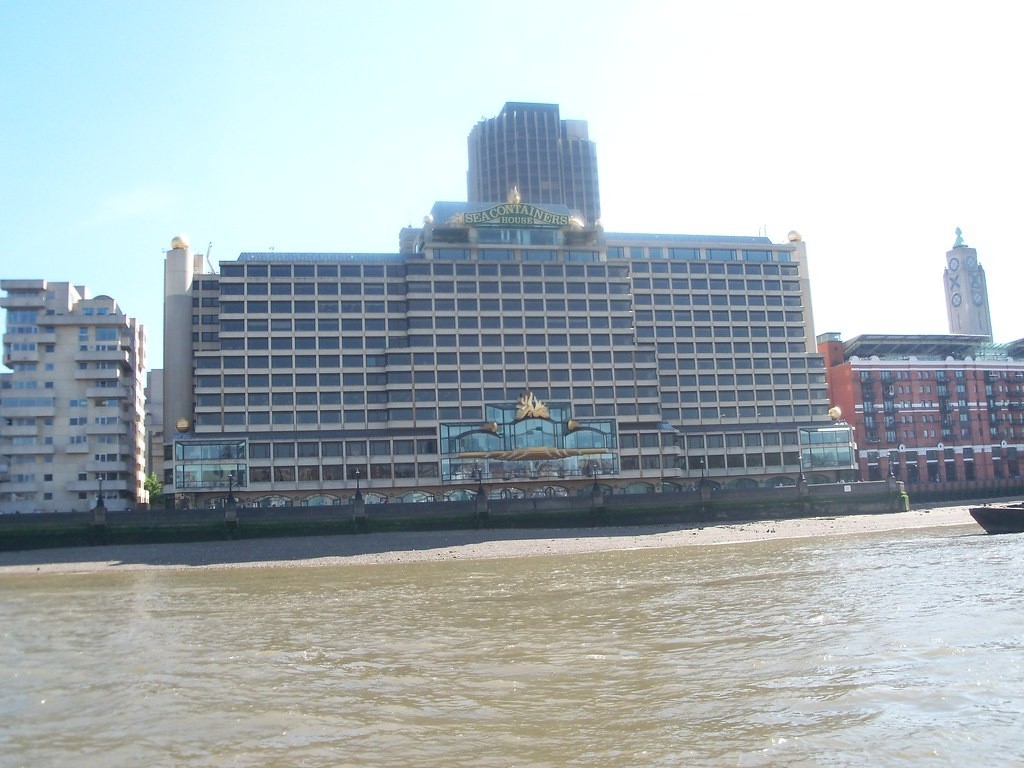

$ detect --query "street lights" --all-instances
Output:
[94,477,106,508]
[226,473,234,504]
[887,452,896,477]
[798,453,807,494]
[593,464,601,491]
[699,458,707,485]
[478,468,486,495]
[355,468,363,500]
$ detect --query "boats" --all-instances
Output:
[969,502,1024,533]
